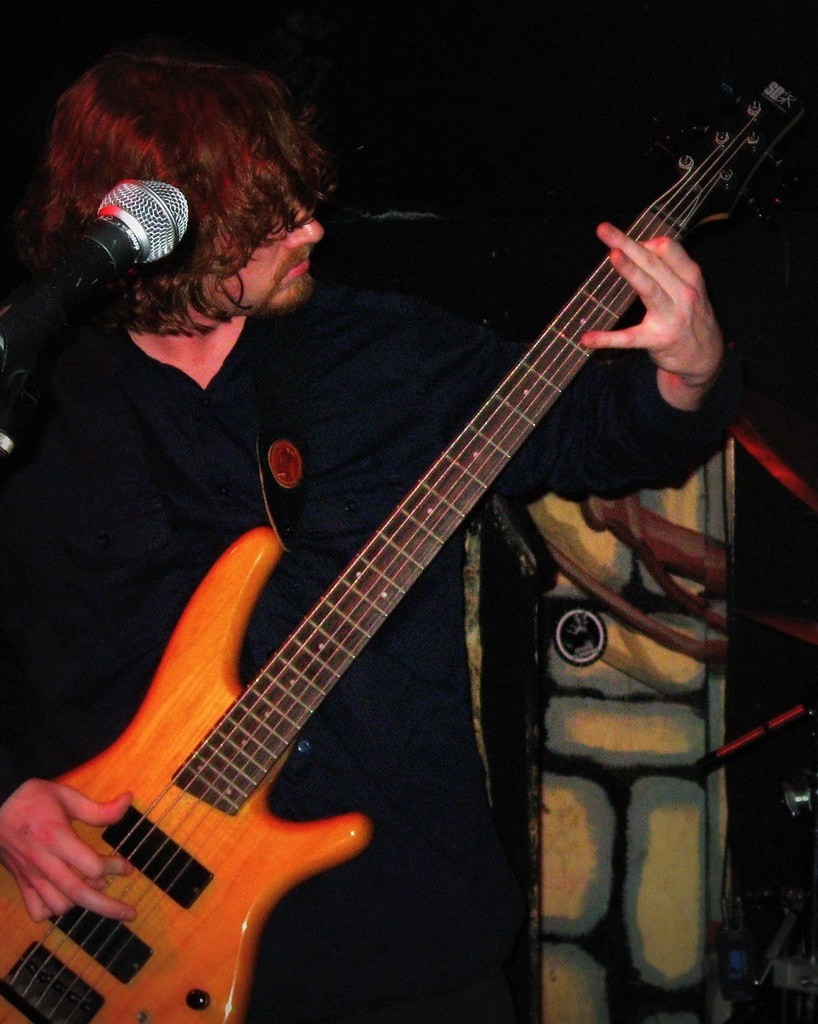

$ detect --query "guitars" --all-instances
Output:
[0,67,811,1024]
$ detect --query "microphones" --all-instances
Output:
[0,178,189,374]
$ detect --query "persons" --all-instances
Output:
[0,46,745,1024]
